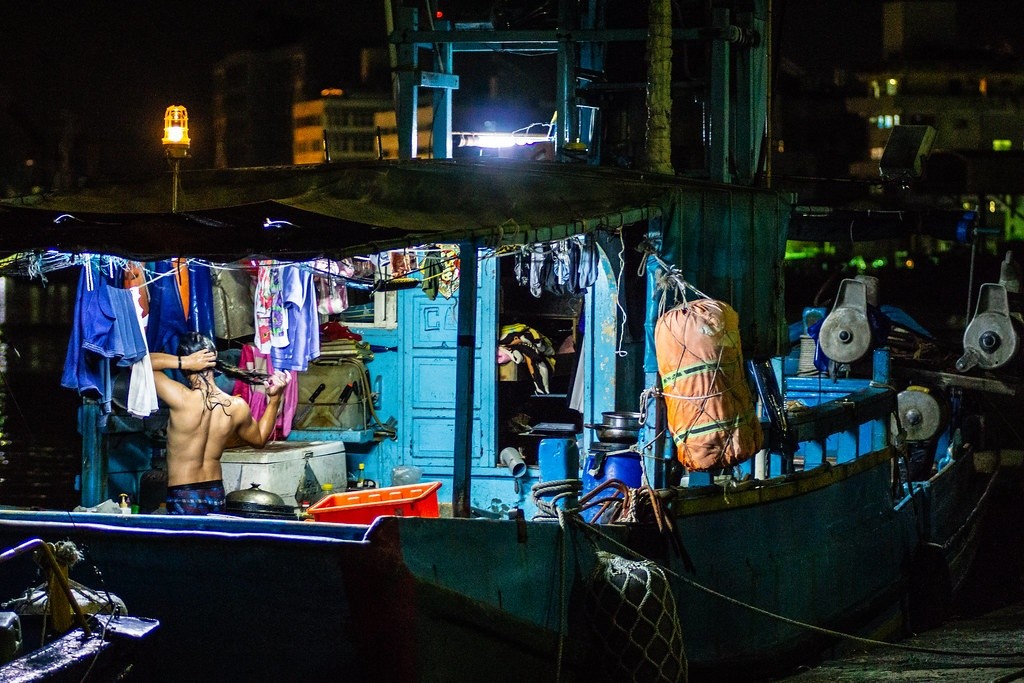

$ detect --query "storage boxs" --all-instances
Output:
[307,481,442,525]
[220,441,347,507]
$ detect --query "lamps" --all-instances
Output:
[161,105,191,211]
[879,124,939,176]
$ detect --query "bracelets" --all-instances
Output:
[177,356,183,369]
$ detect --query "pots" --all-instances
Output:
[222,483,297,521]
[585,411,646,444]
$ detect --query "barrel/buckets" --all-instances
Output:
[579,451,642,524]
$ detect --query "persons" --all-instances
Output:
[128,333,293,519]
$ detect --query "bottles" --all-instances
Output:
[320,484,337,497]
[356,463,365,489]
[300,501,311,513]
[158,503,167,514]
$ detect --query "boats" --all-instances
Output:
[0,158,1024,683]
